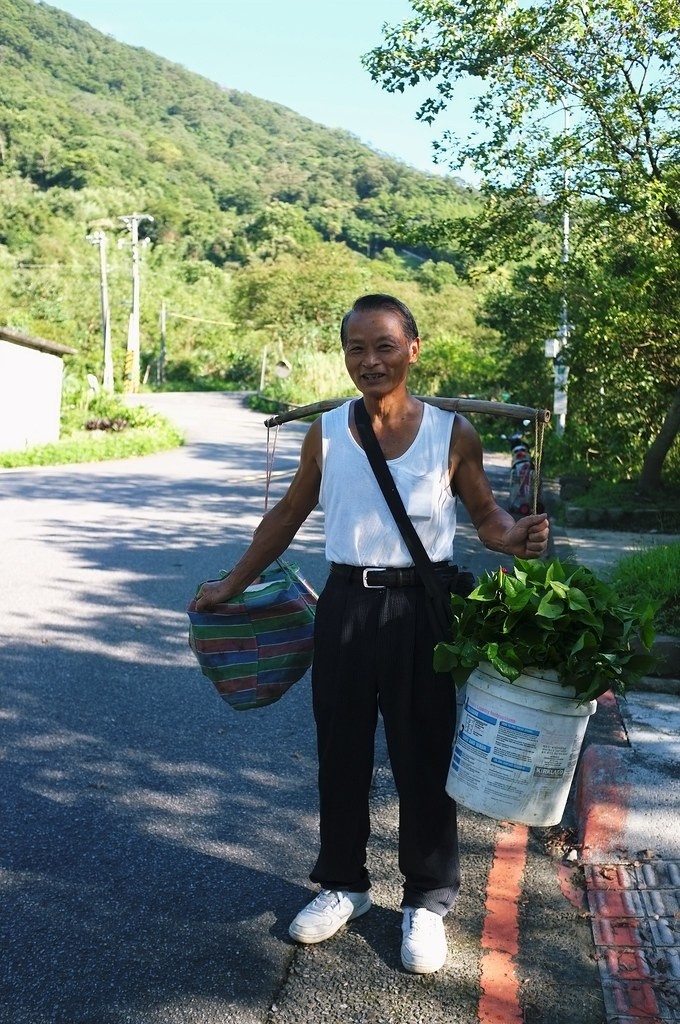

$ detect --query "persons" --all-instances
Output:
[193,289,550,976]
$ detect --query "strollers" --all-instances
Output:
[506,450,545,516]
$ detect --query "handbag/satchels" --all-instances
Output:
[424,586,455,642]
[186,558,319,712]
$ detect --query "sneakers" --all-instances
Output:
[402,907,447,973]
[288,889,371,943]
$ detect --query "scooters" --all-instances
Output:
[501,420,535,482]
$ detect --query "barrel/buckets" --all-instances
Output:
[445,662,597,827]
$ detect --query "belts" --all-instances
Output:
[329,563,459,589]
